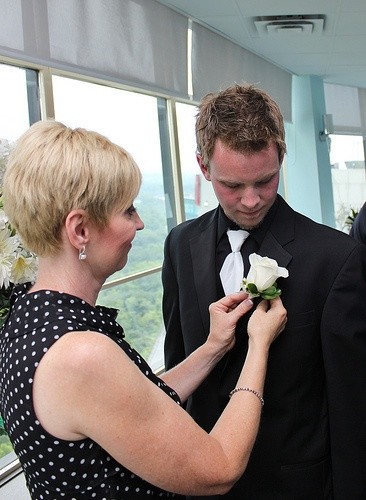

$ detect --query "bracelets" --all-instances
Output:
[229,387,264,409]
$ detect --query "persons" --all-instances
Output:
[0,119,289,500]
[161,83,366,500]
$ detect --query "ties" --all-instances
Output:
[220,230,249,301]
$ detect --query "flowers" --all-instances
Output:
[241,253,289,300]
[0,138,39,328]
